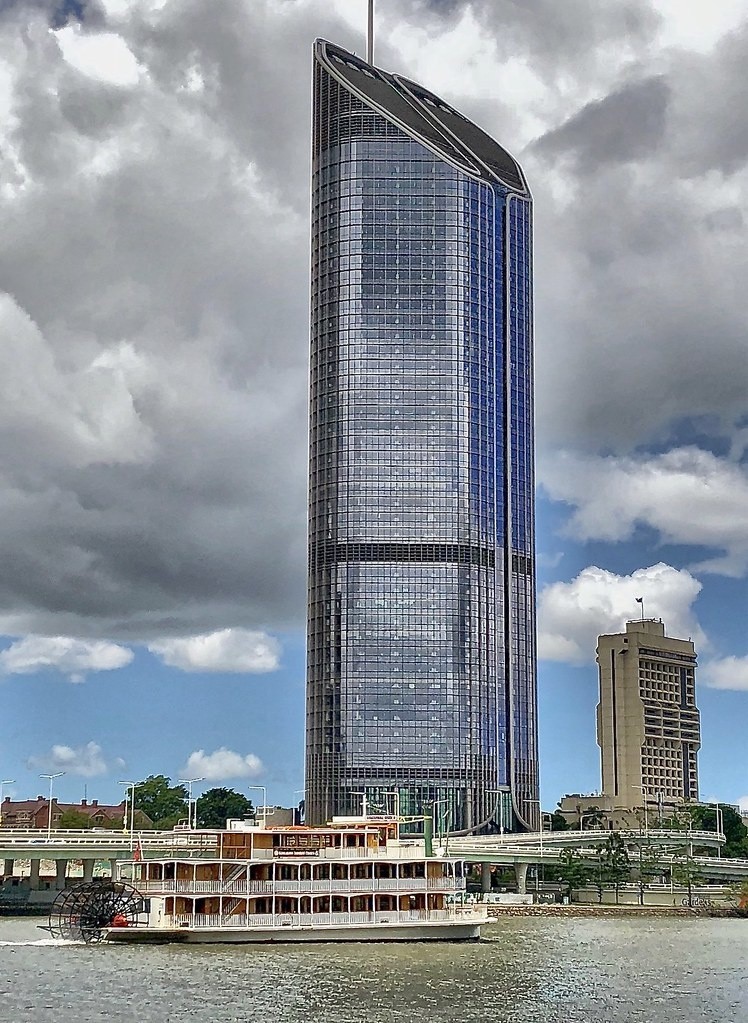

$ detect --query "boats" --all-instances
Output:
[36,829,499,945]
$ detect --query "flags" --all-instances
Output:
[635,597,642,602]
[134,841,140,862]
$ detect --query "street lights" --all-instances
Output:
[708,808,723,833]
[40,772,65,839]
[485,790,503,844]
[677,812,691,830]
[1,780,15,802]
[178,798,196,830]
[581,814,594,831]
[119,781,134,834]
[249,787,266,830]
[348,792,366,820]
[178,777,206,829]
[380,792,399,842]
[125,785,144,830]
[293,790,307,826]
[632,786,647,829]
[433,800,449,838]
[523,800,542,857]
